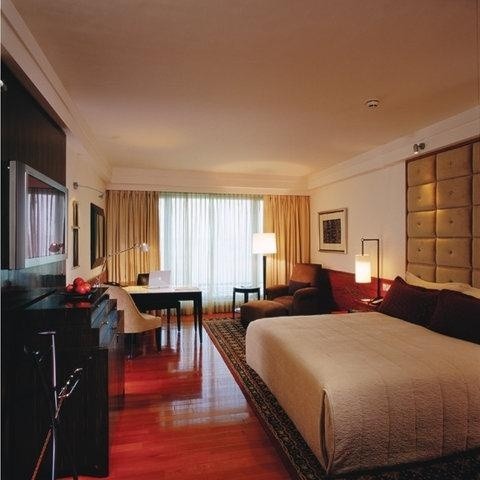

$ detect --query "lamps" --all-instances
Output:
[413,142,426,154]
[101,243,149,267]
[354,239,382,305]
[73,181,104,199]
[253,232,277,300]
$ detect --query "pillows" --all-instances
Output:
[288,279,311,296]
[376,270,479,344]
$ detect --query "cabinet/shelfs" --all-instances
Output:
[24,287,125,479]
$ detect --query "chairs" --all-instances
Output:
[266,262,329,315]
[137,273,181,332]
[106,286,162,357]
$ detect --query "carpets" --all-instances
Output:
[202,315,480,480]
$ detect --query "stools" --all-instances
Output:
[240,300,290,330]
[232,285,260,318]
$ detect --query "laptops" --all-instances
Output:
[142,270,171,288]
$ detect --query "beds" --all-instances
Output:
[246,312,480,480]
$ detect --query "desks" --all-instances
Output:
[121,286,203,344]
[345,295,383,312]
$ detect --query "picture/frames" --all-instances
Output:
[90,202,107,269]
[318,208,348,254]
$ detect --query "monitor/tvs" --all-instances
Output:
[0,161,69,270]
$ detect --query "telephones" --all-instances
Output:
[370,296,384,305]
